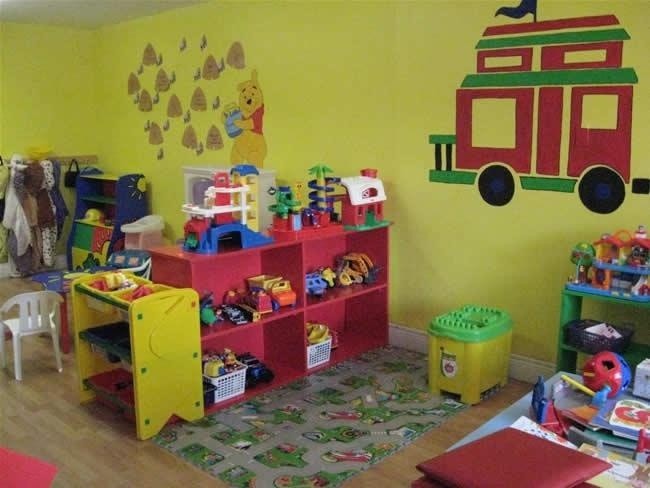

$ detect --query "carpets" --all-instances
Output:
[148,345,506,488]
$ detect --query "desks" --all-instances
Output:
[445,371,649,487]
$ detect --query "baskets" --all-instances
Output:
[307,336,333,368]
[204,359,247,406]
[564,319,633,355]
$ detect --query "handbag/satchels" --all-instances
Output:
[65,171,79,187]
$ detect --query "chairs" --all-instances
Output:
[1,291,66,381]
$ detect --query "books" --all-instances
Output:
[408,398,650,488]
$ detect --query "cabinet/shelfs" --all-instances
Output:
[556,284,650,371]
[66,166,147,272]
[182,165,278,230]
[147,219,395,416]
[69,273,205,440]
